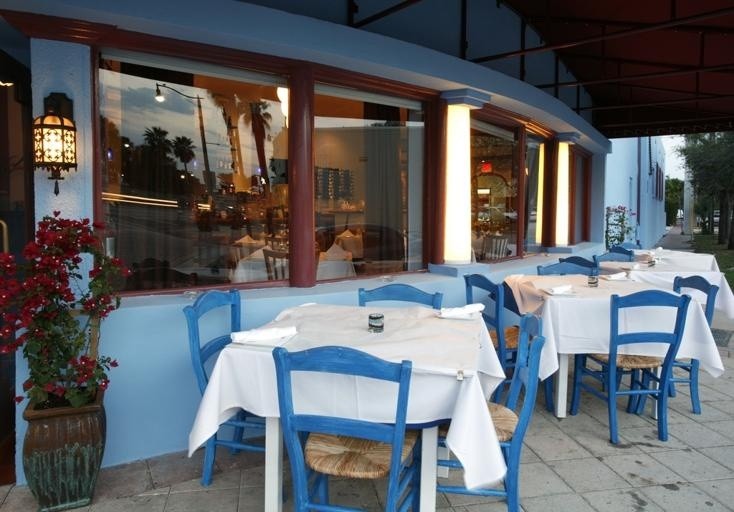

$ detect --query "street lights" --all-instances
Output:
[150,79,215,199]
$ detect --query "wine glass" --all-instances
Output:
[257,223,269,243]
[654,250,664,265]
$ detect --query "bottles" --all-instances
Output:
[339,201,351,210]
[216,133,235,151]
[327,195,333,210]
[215,152,234,170]
[314,195,323,211]
[216,105,232,126]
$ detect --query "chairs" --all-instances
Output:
[191,208,407,283]
[464,241,719,446]
[184,283,546,510]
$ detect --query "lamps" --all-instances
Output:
[31,91,77,194]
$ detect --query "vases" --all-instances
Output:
[22,400,106,512]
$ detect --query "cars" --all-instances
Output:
[713,210,721,227]
[477,204,517,223]
[310,219,433,273]
[195,193,245,232]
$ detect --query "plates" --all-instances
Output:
[435,308,482,320]
[599,274,629,281]
[230,328,299,347]
[621,264,649,271]
[542,286,583,297]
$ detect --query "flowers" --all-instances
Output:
[0,211,131,407]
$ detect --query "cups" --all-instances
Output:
[588,273,599,287]
[368,313,384,333]
[648,256,656,266]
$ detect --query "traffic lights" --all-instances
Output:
[258,176,269,186]
